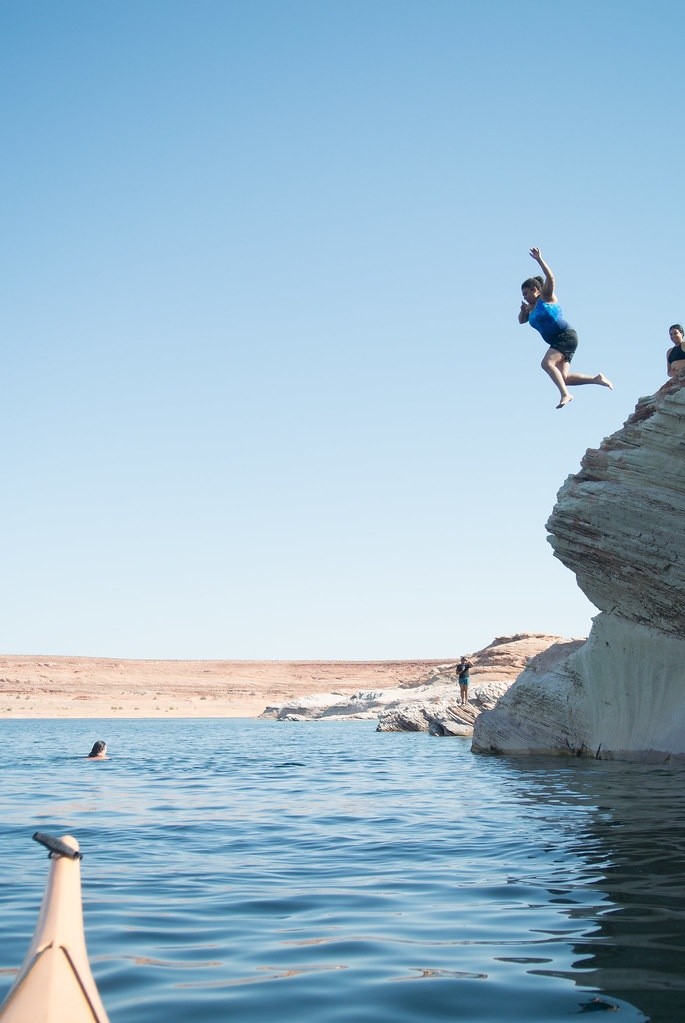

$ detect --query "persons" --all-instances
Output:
[667,324,685,378]
[518,248,612,409]
[456,657,474,703]
[89,741,107,756]
